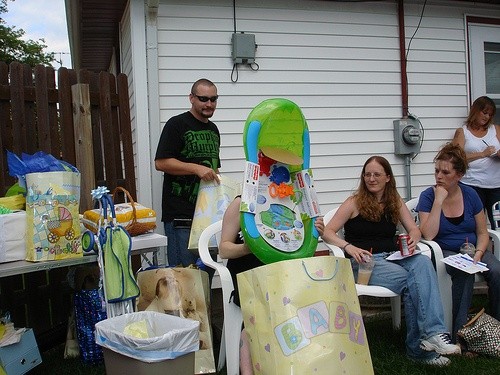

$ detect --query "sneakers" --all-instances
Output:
[407,352,451,366]
[420,333,462,356]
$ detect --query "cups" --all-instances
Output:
[356,257,376,285]
[460,243,476,258]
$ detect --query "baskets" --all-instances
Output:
[112,186,156,236]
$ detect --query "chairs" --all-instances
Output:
[197,198,500,375]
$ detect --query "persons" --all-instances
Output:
[322,156,464,367]
[219,195,325,375]
[453,96,500,255]
[415,142,500,358]
[154,78,223,313]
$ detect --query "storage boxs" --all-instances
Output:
[0,328,42,375]
[0,210,27,263]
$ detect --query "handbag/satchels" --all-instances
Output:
[457,308,500,357]
[135,265,217,374]
[236,256,374,375]
[24,170,84,262]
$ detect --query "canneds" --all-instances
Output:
[398,233,414,257]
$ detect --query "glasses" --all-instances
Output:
[193,93,218,102]
[363,173,387,179]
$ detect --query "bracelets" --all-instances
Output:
[344,243,351,251]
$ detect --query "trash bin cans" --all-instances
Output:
[94,311,201,375]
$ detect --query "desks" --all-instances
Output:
[0,233,167,321]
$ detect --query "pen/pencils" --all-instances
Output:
[481,140,489,147]
[461,256,486,266]
